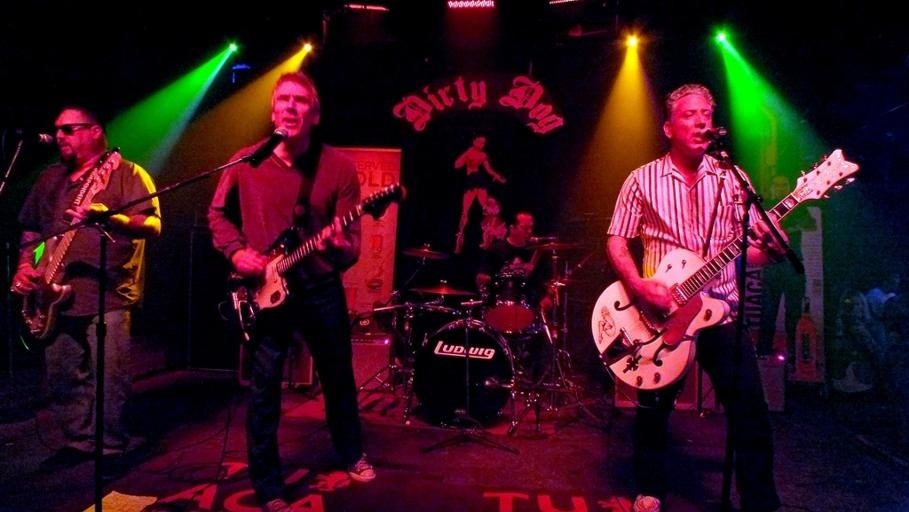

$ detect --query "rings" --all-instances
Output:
[11,290,15,292]
[15,281,22,287]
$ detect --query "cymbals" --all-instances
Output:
[412,287,475,296]
[405,246,450,262]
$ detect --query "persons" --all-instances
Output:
[476,211,560,384]
[10,103,162,459]
[208,72,376,512]
[606,84,790,510]
[452,130,508,253]
[823,266,909,405]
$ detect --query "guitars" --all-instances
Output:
[591,146,860,392]
[223,182,408,346]
[11,147,125,356]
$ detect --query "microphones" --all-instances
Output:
[2,131,54,144]
[250,127,288,165]
[702,127,727,140]
[529,236,557,244]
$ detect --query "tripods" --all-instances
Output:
[355,252,608,455]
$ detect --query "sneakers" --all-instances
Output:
[349,451,376,482]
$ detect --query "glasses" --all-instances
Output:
[49,123,92,134]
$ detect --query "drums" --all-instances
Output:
[412,317,518,419]
[481,269,539,335]
